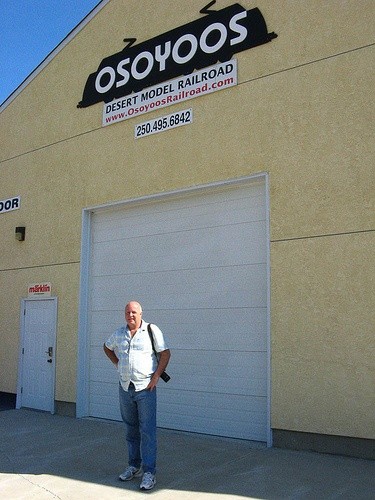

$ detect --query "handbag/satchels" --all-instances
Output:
[155,362,173,384]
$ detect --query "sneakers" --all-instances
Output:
[118,465,143,481]
[139,471,157,490]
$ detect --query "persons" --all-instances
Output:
[102,301,170,491]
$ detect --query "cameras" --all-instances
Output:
[151,367,170,384]
